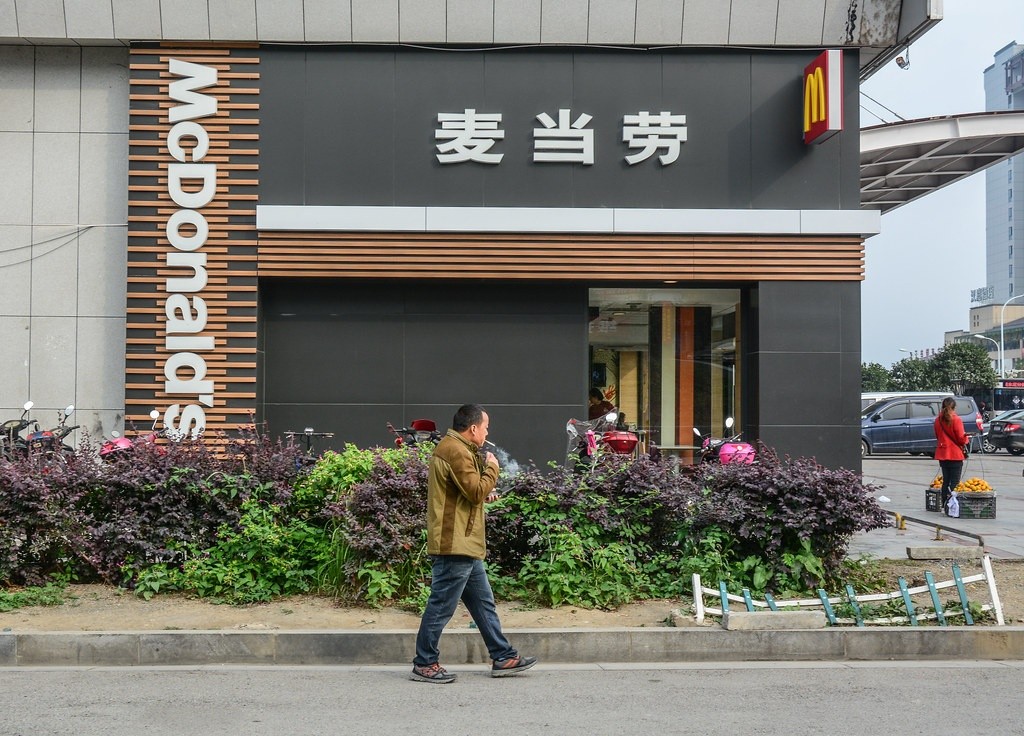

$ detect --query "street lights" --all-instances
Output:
[973,334,1000,378]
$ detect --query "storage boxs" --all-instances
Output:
[925,488,944,512]
[953,489,997,519]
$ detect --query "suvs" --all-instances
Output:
[859,394,984,458]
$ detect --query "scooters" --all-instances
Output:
[284,426,335,480]
[566,406,639,488]
[692,416,756,469]
[0,401,39,464]
[98,409,171,469]
[28,405,80,468]
[386,416,446,453]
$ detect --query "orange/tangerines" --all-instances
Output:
[930,476,992,492]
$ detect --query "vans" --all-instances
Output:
[861,391,955,416]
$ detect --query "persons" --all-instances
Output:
[588,387,615,420]
[409,402,538,684]
[616,412,628,431]
[977,401,989,421]
[933,397,971,511]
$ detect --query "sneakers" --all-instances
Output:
[410,662,457,683]
[492,652,538,677]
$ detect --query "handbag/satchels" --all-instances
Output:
[962,444,969,459]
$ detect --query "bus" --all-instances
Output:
[954,376,1024,421]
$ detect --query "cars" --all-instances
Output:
[971,419,999,454]
[988,410,1024,456]
